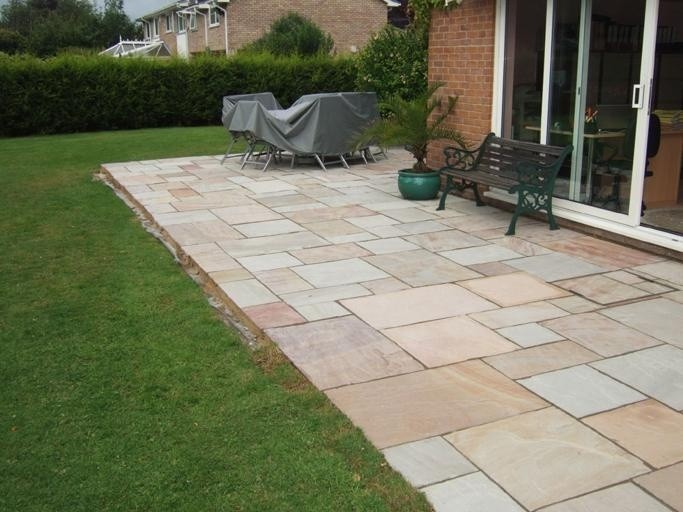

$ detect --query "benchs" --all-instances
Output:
[435,132,574,236]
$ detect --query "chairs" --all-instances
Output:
[585,114,661,217]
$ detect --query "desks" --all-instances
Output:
[524,125,683,211]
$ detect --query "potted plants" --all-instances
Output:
[342,81,477,201]
